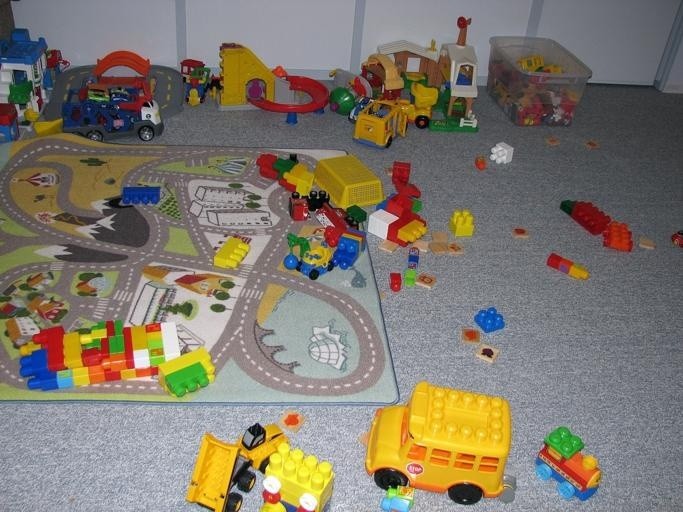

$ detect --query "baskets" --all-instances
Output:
[314,155,386,210]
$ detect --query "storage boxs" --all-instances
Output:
[486,36,592,127]
